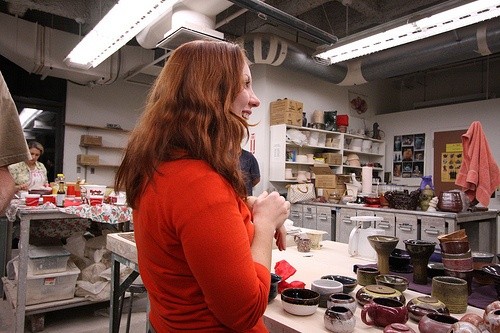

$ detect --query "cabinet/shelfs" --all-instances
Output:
[270,123,386,183]
[289,203,465,246]
[79,144,126,168]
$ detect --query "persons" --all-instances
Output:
[113,40,291,333]
[0,69,34,214]
[238,145,261,196]
[8,141,48,188]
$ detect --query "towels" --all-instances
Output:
[455,120,500,207]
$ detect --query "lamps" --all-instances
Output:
[310,0,500,65]
[63,0,179,70]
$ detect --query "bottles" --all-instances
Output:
[394,133,425,179]
[286,128,341,203]
[313,109,324,123]
[303,111,307,127]
[417,175,464,213]
[55,174,66,207]
[67,232,86,258]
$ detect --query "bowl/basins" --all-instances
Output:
[345,138,391,206]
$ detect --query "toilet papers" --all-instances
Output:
[362,166,373,194]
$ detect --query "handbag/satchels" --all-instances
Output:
[285,183,316,203]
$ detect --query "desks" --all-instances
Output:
[0,185,133,333]
[106,232,500,333]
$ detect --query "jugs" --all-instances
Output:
[348,216,383,262]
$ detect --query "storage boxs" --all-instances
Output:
[317,153,342,165]
[77,154,99,164]
[13,260,81,306]
[270,98,303,126]
[80,134,102,146]
[27,245,71,274]
[315,175,345,200]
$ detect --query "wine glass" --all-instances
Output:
[346,138,352,149]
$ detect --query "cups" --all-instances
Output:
[268,227,500,333]
[16,180,129,206]
